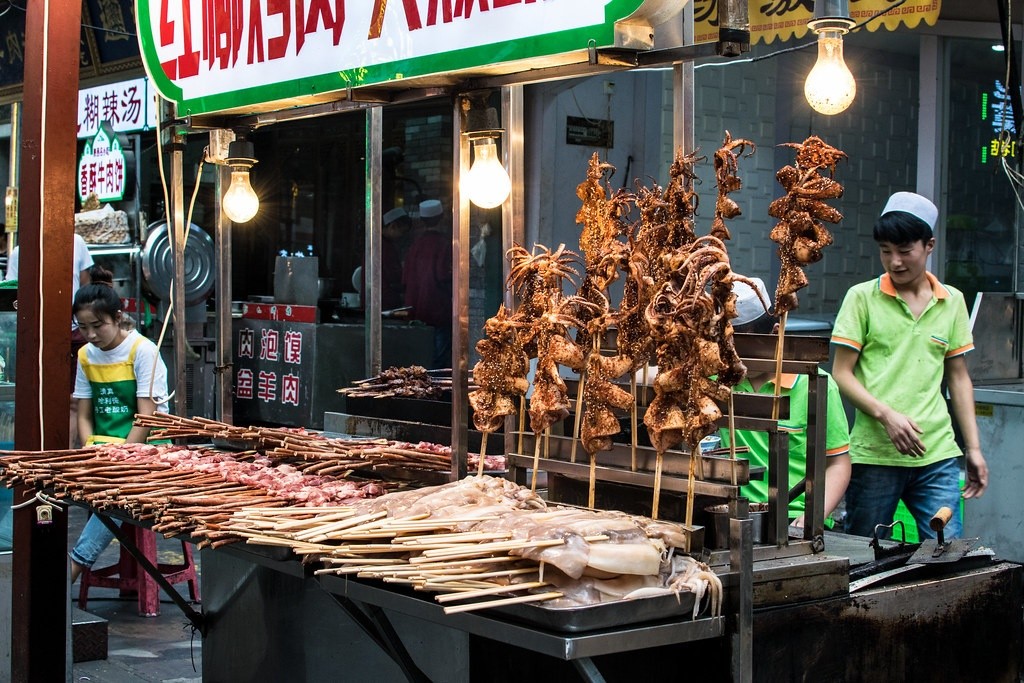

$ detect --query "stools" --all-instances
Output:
[79,506,198,616]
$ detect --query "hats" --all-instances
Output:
[730,272,772,326]
[880,192,938,232]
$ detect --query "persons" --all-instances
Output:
[68,266,172,585]
[830,192,989,543]
[709,277,852,529]
[360,206,402,320]
[5,233,94,449]
[402,199,451,412]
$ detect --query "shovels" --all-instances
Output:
[906,506,981,565]
